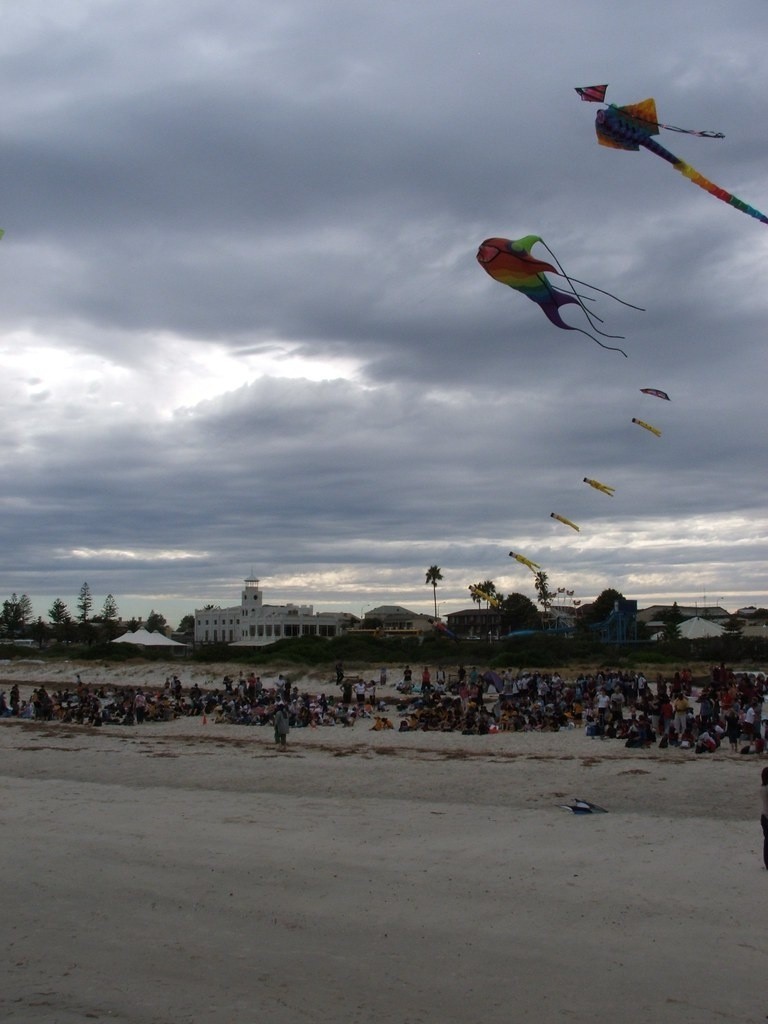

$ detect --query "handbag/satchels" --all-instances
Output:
[143,699,147,706]
[93,717,102,726]
[602,712,613,722]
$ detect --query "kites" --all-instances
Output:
[427,388,672,644]
[595,99,768,223]
[577,85,727,141]
[477,235,646,359]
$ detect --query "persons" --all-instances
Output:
[1,659,768,761]
[760,766,768,871]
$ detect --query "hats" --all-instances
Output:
[138,690,144,695]
[374,715,380,719]
[522,672,529,675]
[639,715,645,721]
